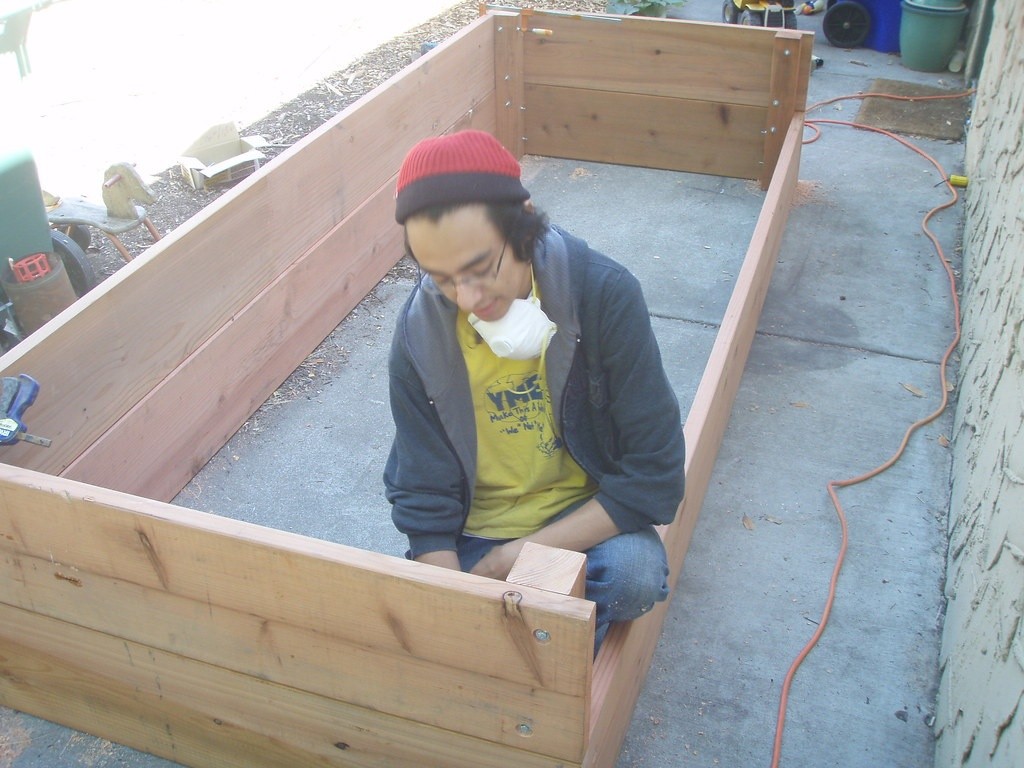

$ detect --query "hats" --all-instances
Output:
[395,130,530,224]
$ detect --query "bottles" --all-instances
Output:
[948,50,965,72]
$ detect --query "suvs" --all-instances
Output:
[723,0,797,31]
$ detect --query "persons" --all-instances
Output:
[383,129,686,631]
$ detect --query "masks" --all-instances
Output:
[468,298,557,360]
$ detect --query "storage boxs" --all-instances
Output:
[180,122,271,190]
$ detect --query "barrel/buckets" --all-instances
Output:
[898,0,970,72]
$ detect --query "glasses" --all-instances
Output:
[418,228,510,296]
